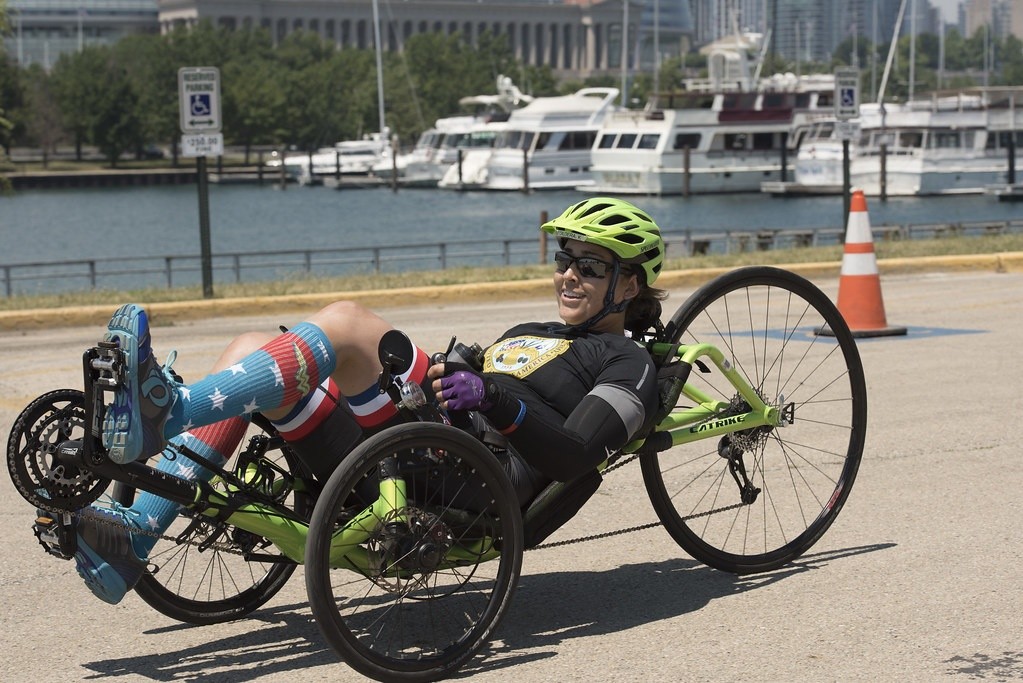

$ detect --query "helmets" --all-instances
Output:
[540,198,665,286]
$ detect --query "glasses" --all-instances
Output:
[555,250,633,279]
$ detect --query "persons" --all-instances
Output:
[34,196,667,606]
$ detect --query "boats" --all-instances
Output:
[266,1,1023,198]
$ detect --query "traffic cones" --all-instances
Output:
[814,191,907,338]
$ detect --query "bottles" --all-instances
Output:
[447,342,485,373]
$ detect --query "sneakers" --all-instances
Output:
[102,301,180,465]
[37,488,160,606]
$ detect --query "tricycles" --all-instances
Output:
[7,267,867,682]
